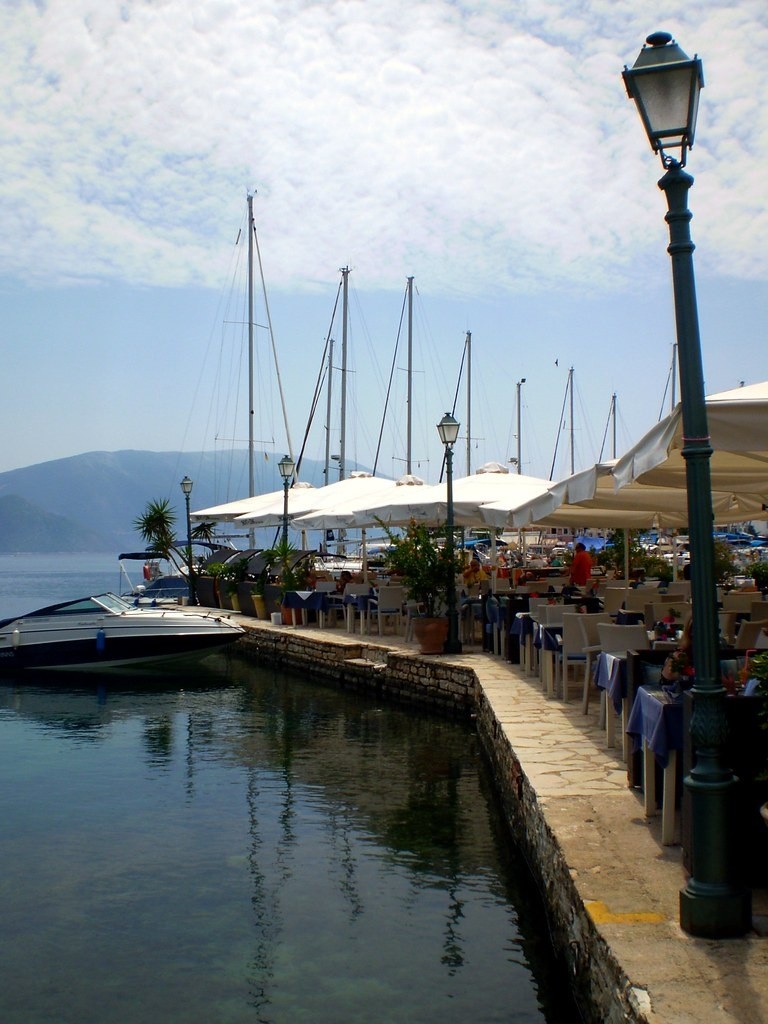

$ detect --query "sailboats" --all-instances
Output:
[106,193,768,610]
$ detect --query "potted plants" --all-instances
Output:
[217,558,250,612]
[371,512,470,656]
[249,565,271,619]
[261,539,316,625]
[747,651,768,828]
[206,562,229,609]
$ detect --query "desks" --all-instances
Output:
[595,651,629,763]
[534,627,563,692]
[630,581,669,594]
[343,594,378,636]
[282,590,335,629]
[624,685,768,846]
[509,612,538,677]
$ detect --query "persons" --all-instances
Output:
[568,542,593,586]
[463,558,488,585]
[659,611,694,686]
[338,571,359,592]
[549,554,561,566]
[515,555,523,567]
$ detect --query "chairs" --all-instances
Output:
[315,570,768,730]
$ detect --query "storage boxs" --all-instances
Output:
[271,613,283,625]
[178,596,189,606]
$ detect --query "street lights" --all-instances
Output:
[277,454,296,574]
[436,412,463,654]
[620,29,758,939]
[180,475,199,607]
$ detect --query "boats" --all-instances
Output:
[0,553,246,695]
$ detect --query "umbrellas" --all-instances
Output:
[188,381,768,616]
